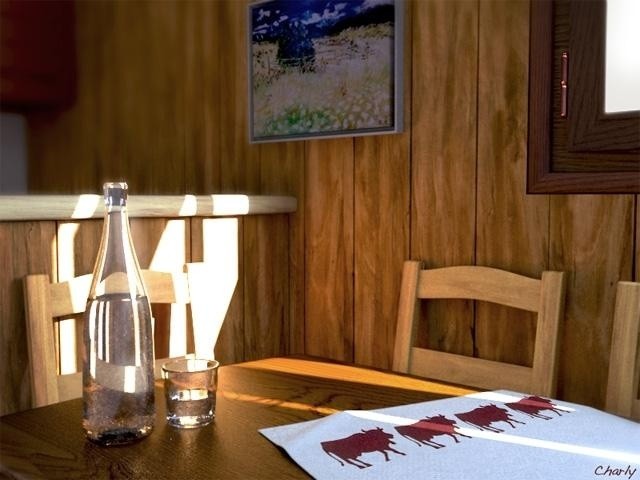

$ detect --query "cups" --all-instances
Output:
[161,357,220,430]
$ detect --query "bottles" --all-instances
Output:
[81,181,156,448]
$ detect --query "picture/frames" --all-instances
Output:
[246,0,405,145]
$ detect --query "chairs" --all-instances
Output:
[603,280,640,424]
[391,259,567,399]
[22,260,209,410]
